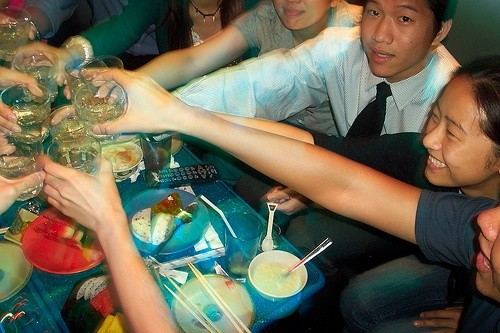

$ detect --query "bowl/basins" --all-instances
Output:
[248,250,308,304]
[106,140,144,182]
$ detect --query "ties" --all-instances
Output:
[339,81,393,164]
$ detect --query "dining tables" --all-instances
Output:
[0,141,326,333]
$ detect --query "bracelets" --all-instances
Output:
[66,36,94,67]
[24,17,40,39]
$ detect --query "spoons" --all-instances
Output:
[150,202,199,258]
[262,202,279,252]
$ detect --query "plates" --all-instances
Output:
[122,187,209,256]
[134,134,185,157]
[172,274,255,333]
[61,269,132,333]
[22,206,105,275]
[0,241,33,303]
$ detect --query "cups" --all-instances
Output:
[260,222,282,253]
[47,56,127,144]
[0,82,52,145]
[13,48,59,103]
[223,211,264,278]
[140,130,173,171]
[47,134,102,176]
[0,6,33,62]
[0,132,44,202]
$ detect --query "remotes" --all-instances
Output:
[144,164,221,188]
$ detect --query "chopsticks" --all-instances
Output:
[188,261,253,333]
[164,275,223,333]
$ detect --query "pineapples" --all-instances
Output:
[93,312,133,333]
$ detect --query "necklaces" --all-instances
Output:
[189,0,221,23]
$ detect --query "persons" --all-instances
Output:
[0,0,500,333]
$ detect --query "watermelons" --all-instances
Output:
[90,285,120,316]
[34,213,93,250]
